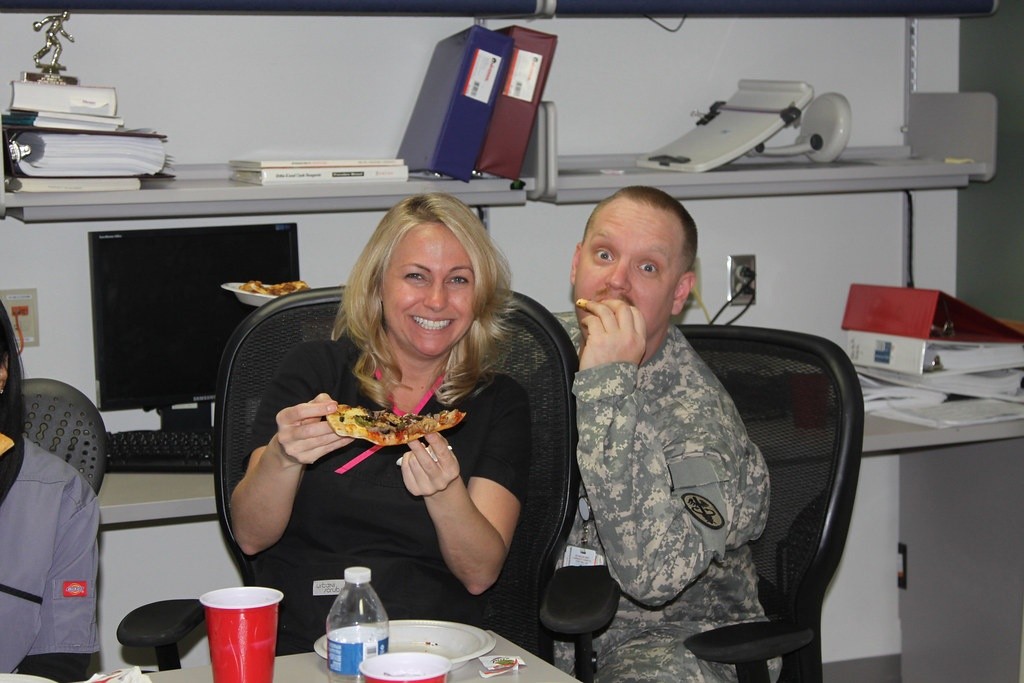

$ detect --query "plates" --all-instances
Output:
[222,281,277,307]
[313,618,498,670]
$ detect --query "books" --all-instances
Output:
[229,159,409,186]
[2,79,176,192]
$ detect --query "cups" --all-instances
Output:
[358,650,451,683]
[199,585,283,683]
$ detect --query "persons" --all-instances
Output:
[552,185,770,683]
[0,300,100,683]
[230,193,533,657]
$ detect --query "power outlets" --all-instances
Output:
[727,254,757,308]
[0,289,40,347]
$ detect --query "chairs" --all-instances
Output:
[19,378,108,496]
[114,284,623,682]
[681,324,863,682]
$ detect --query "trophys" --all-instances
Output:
[20,10,78,85]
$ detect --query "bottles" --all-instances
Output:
[326,567,389,683]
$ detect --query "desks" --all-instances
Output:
[132,629,579,683]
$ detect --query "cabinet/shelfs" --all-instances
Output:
[0,0,1024,682]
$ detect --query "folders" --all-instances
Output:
[841,284,1024,343]
[396,25,515,184]
[475,26,558,181]
[845,329,1024,376]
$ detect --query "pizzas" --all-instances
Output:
[577,298,589,309]
[325,403,467,445]
[250,280,310,295]
